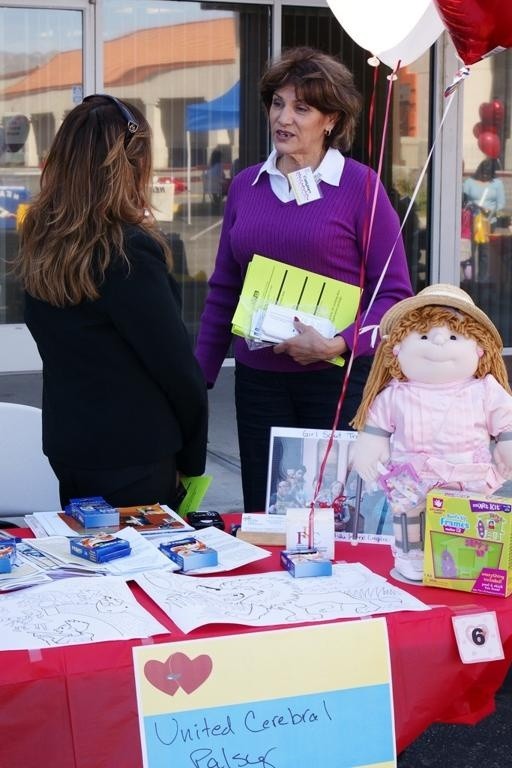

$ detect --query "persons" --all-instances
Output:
[11,94,208,516]
[268,464,354,536]
[346,282,511,582]
[465,158,506,287]
[195,43,414,511]
[201,148,227,218]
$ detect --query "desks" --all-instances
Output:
[0,320,42,405]
[2,503,509,768]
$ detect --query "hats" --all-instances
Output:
[378,283,502,353]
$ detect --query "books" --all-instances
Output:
[230,253,364,370]
[2,502,291,595]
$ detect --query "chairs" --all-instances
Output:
[0,399,62,514]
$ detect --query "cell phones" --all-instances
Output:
[186,509,226,532]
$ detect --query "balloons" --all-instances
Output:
[376,0,445,81]
[327,0,435,68]
[432,0,512,66]
[472,100,512,158]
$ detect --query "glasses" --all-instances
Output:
[84,92,139,151]
